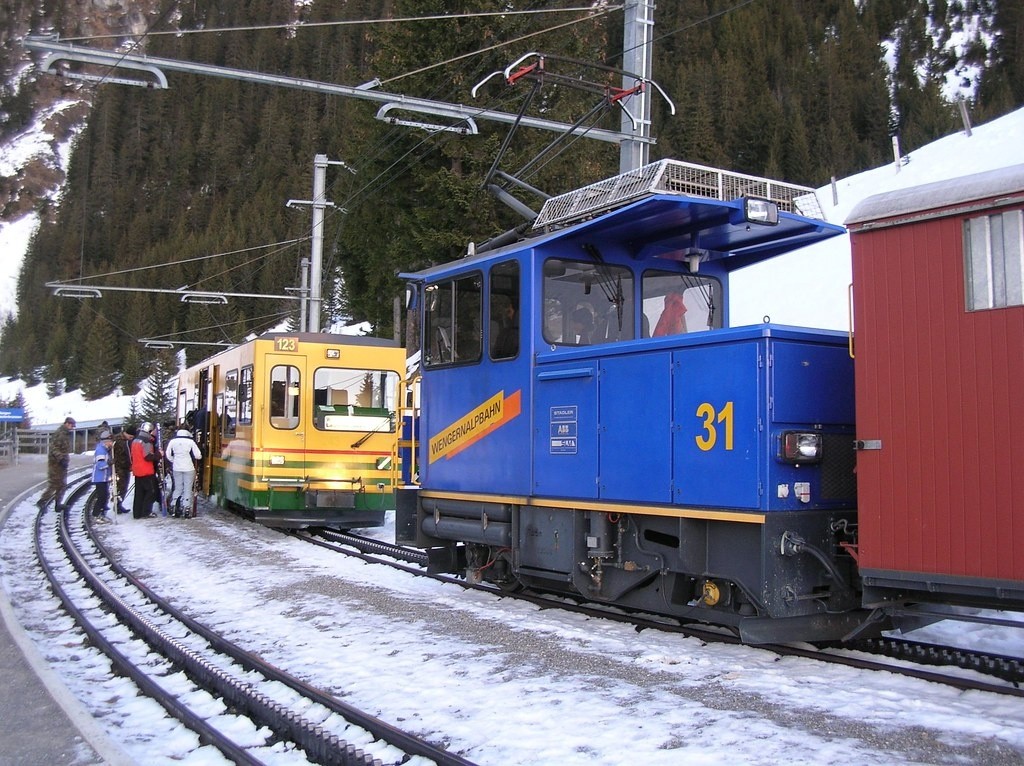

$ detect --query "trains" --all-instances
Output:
[164,293,407,533]
[394,49,1024,646]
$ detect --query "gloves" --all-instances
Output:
[60,458,68,467]
[107,459,116,465]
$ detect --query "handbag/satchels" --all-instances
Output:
[164,474,172,491]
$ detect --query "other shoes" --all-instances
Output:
[134,511,158,519]
[112,505,130,513]
[185,510,192,518]
[55,504,70,512]
[37,499,47,513]
[171,507,178,516]
[94,514,112,523]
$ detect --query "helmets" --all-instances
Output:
[140,422,154,432]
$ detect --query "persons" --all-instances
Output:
[218,427,252,502]
[91,398,207,524]
[569,294,650,346]
[37,416,76,513]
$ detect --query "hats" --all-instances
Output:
[126,425,137,435]
[572,307,593,324]
[100,431,113,441]
[67,417,76,427]
